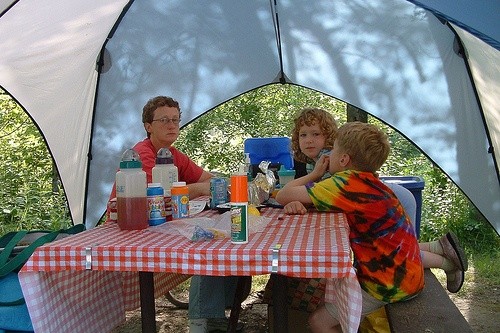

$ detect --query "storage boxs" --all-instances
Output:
[243,138,294,172]
[378,177,424,237]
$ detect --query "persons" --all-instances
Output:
[290,108,469,293]
[275,121,425,333]
[110,95,251,333]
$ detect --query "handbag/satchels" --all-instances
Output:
[0,224,87,278]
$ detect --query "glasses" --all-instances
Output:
[150,117,181,126]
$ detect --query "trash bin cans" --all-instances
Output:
[377,176,424,244]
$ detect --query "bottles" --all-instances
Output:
[277,165,296,189]
[171,182,190,220]
[146,182,166,226]
[115,160,149,230]
[151,151,179,216]
[230,175,249,245]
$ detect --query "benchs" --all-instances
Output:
[383,267,473,333]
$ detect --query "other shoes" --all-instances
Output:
[439,232,467,271]
[447,259,464,293]
[188,317,242,331]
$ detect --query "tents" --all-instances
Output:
[0,0,500,237]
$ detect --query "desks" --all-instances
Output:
[18,194,363,333]
